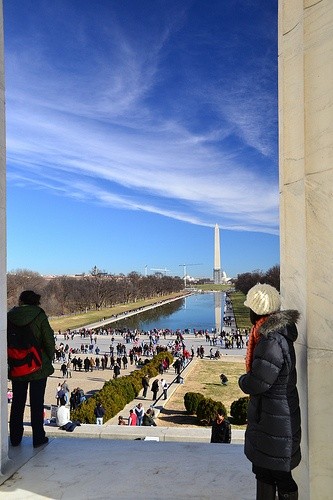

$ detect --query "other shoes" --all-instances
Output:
[11,439,20,446]
[33,437,49,448]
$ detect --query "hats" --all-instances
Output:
[21,290,41,306]
[244,283,281,315]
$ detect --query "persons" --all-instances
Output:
[151,378,159,401]
[118,403,158,440]
[60,359,73,378]
[175,373,183,383]
[94,402,106,425]
[56,380,70,407]
[7,389,13,403]
[161,379,167,400]
[56,399,72,427]
[142,374,150,399]
[238,284,302,500]
[6,290,55,448]
[52,327,249,374]
[114,363,121,379]
[225,321,232,327]
[219,374,228,386]
[211,409,231,444]
[69,387,86,412]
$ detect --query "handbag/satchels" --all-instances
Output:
[55,395,58,398]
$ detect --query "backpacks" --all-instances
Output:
[7,324,42,378]
